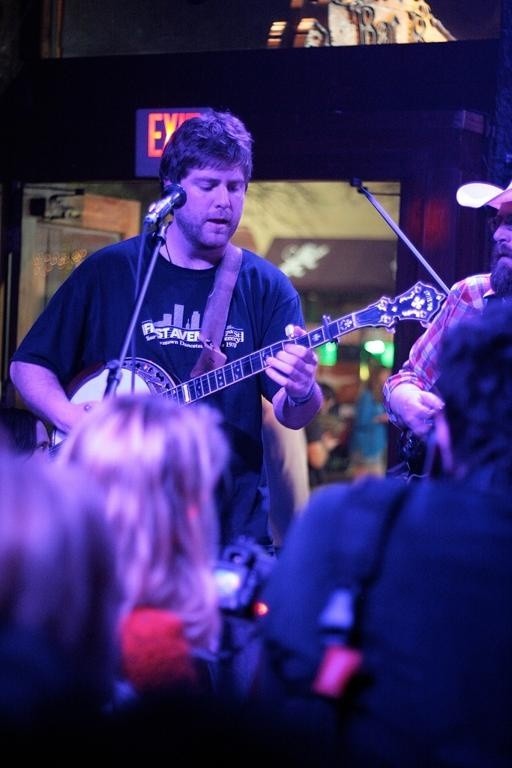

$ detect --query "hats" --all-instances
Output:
[457,176,512,213]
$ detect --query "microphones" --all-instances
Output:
[143,184,187,228]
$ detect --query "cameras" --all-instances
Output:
[203,545,262,618]
